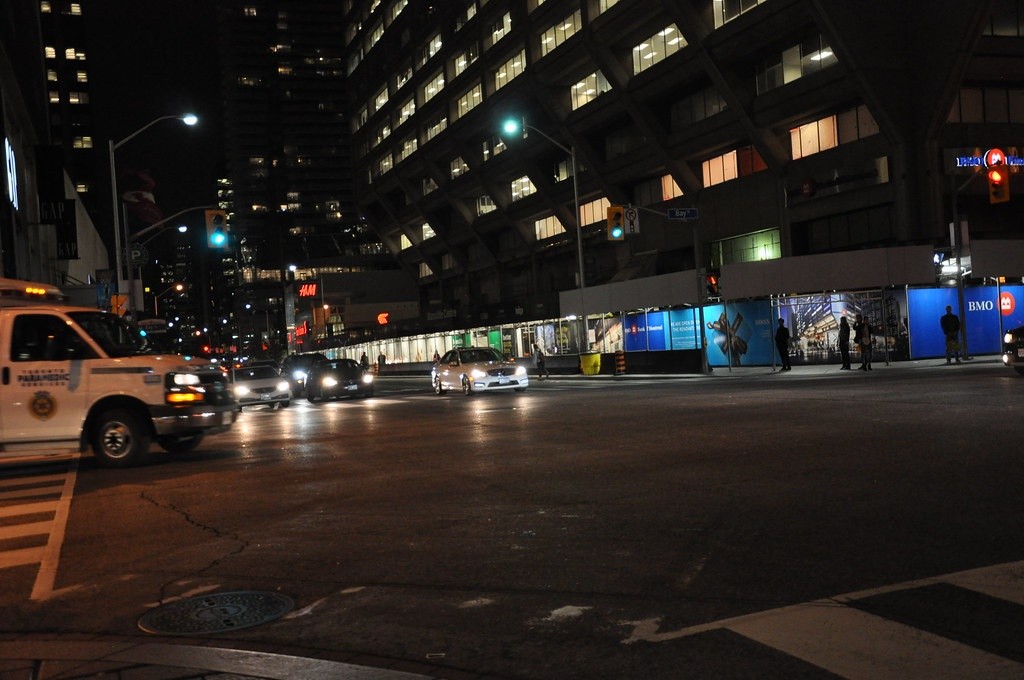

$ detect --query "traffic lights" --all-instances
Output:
[205,208,228,248]
[606,207,623,240]
[111,294,128,317]
[204,346,209,352]
[706,268,719,296]
[987,165,1008,204]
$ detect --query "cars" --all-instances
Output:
[306,358,373,402]
[228,365,291,409]
[1002,325,1024,374]
[431,344,530,395]
[247,361,285,376]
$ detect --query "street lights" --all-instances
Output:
[289,265,328,352]
[109,114,199,295]
[138,224,187,317]
[504,120,591,355]
[323,304,341,344]
[154,284,183,315]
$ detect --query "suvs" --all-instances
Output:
[281,352,330,398]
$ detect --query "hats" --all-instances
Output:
[778,318,784,323]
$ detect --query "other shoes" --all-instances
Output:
[786,367,791,370]
[840,364,850,370]
[868,365,872,370]
[947,360,951,364]
[956,360,960,365]
[863,366,867,371]
[781,366,786,370]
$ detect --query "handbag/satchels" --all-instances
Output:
[854,337,859,343]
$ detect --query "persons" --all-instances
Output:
[940,305,961,365]
[532,344,549,379]
[377,351,386,369]
[549,343,558,355]
[853,315,874,371]
[775,318,792,371]
[836,317,850,370]
[360,352,369,375]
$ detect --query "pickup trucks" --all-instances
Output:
[0,279,240,468]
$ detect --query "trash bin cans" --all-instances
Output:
[579,353,601,375]
